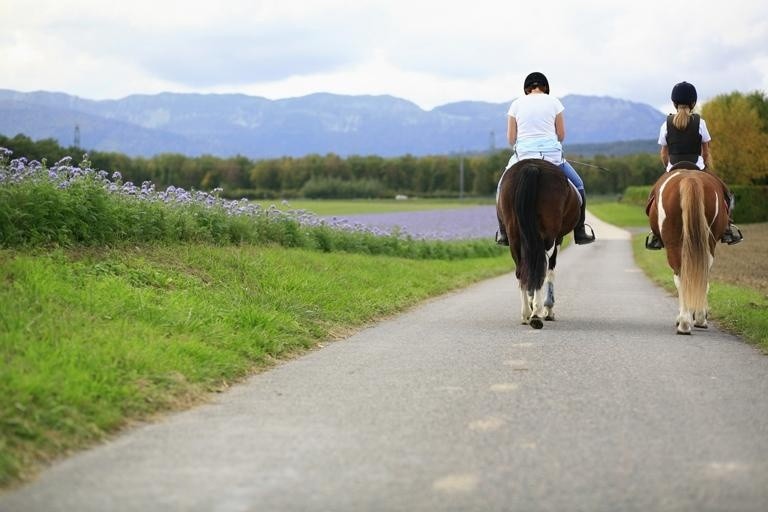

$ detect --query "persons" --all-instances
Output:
[494,71,596,246]
[645,80,744,249]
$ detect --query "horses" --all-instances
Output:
[648,168,730,336]
[494,155,584,330]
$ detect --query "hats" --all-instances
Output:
[671,81,697,104]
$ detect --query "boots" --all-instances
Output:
[648,236,662,247]
[497,234,508,242]
[574,226,595,244]
[721,234,740,243]
